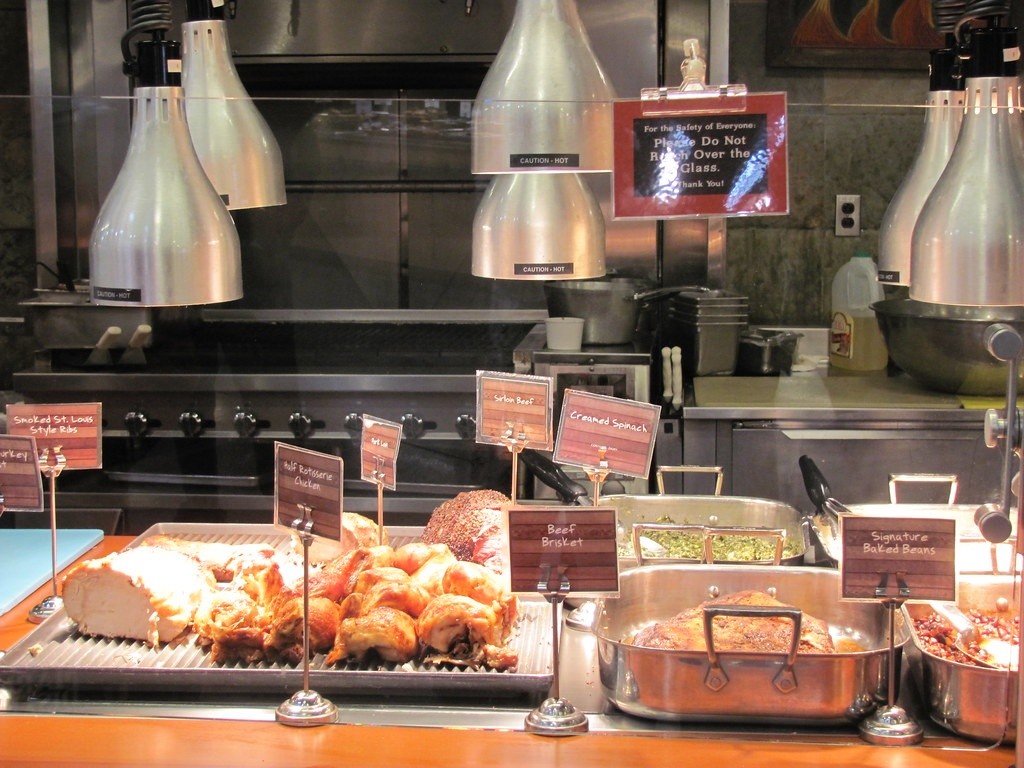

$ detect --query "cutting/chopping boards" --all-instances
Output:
[692,374,963,409]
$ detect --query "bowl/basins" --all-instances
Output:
[868,300,1022,395]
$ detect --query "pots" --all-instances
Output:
[544,281,709,345]
[563,465,1018,741]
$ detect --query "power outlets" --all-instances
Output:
[836,194,861,236]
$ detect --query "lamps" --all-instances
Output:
[876,0,986,287]
[472,175,607,282]
[89,0,244,307]
[471,1,617,175]
[176,0,289,209]
[910,1,1024,308]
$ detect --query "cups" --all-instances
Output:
[545,318,585,350]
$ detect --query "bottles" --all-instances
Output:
[828,257,890,372]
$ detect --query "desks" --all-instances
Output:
[0,536,1019,768]
